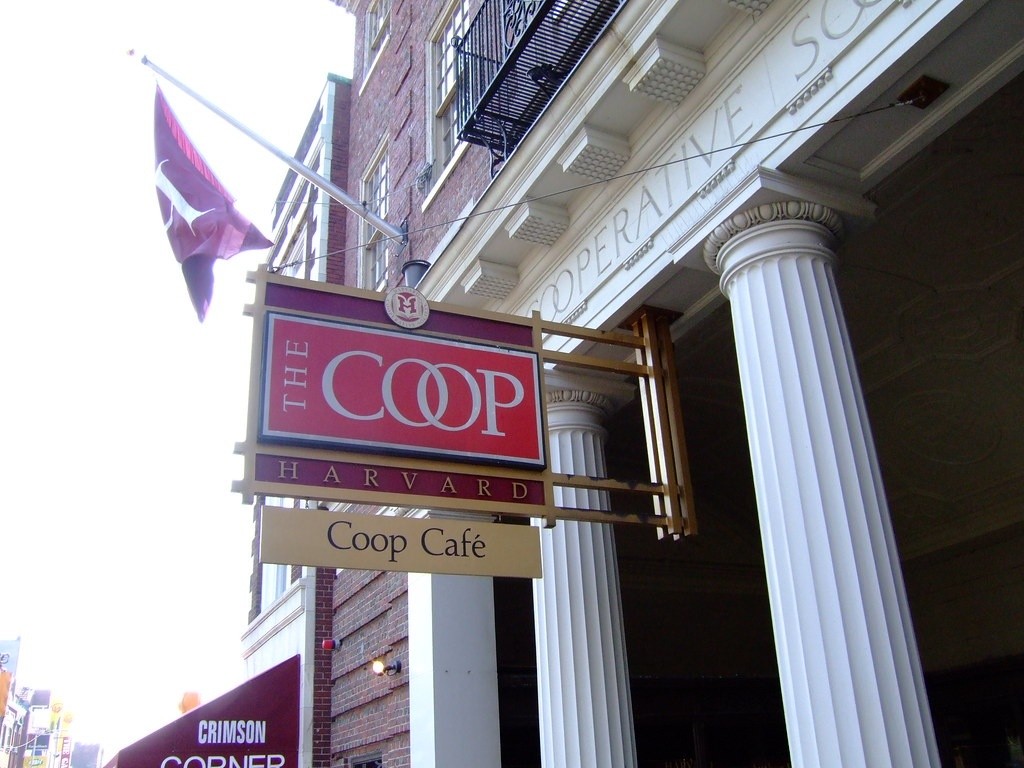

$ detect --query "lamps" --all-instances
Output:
[372,658,402,676]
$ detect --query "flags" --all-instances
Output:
[154,84,274,323]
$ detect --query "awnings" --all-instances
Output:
[103,653,299,768]
